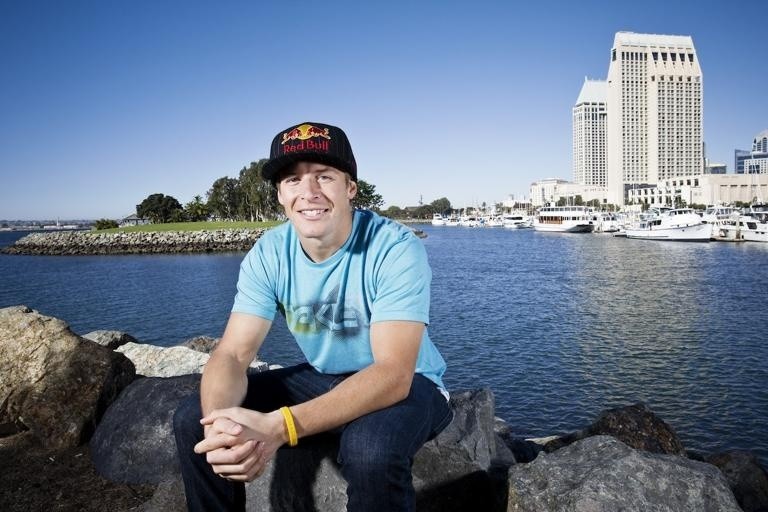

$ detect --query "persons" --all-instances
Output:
[173,121,453,512]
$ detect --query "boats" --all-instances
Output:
[432,205,768,242]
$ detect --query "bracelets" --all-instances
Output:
[280,406,298,447]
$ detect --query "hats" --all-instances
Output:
[260,121,357,183]
